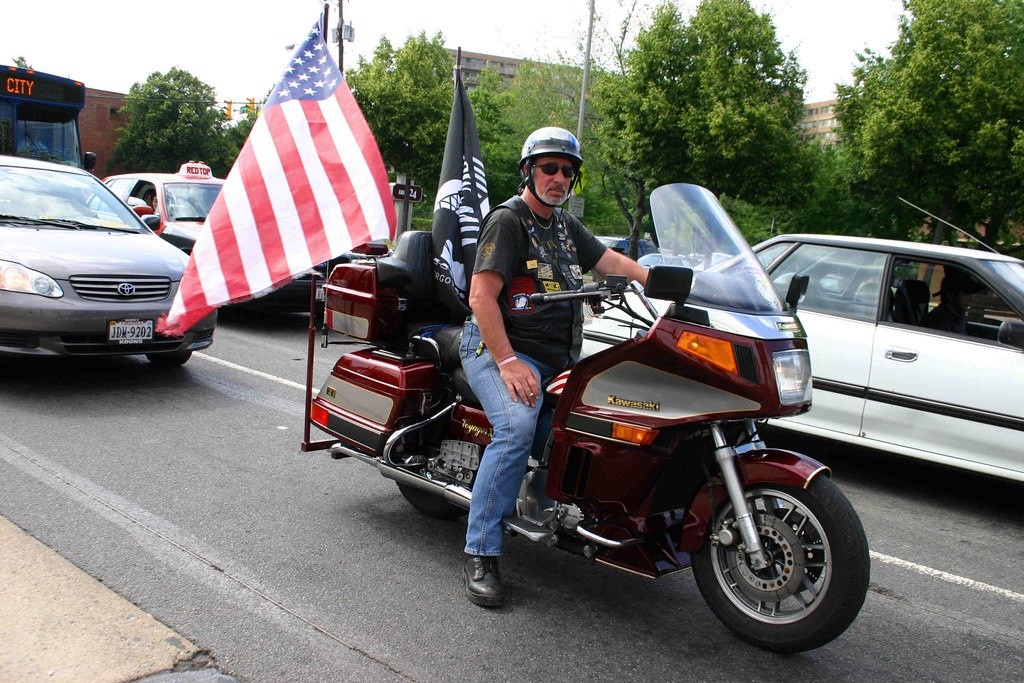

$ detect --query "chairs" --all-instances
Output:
[853,282,895,323]
[893,279,932,326]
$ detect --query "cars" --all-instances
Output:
[581,193,1023,485]
[86,158,352,327]
[0,156,220,370]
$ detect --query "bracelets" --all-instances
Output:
[498,355,518,370]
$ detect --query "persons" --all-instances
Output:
[17,125,50,160]
[925,269,990,343]
[150,191,159,213]
[458,123,656,611]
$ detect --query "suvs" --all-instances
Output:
[592,233,659,263]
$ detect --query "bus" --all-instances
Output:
[0,64,95,176]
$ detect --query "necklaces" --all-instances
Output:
[521,194,555,230]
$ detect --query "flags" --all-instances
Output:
[429,68,493,318]
[154,15,398,337]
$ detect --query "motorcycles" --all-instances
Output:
[156,4,869,656]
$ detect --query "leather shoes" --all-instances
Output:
[460,555,505,607]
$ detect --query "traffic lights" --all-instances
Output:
[223,99,232,121]
[243,97,255,114]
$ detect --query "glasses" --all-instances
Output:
[525,162,577,179]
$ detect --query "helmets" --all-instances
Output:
[517,126,582,171]
